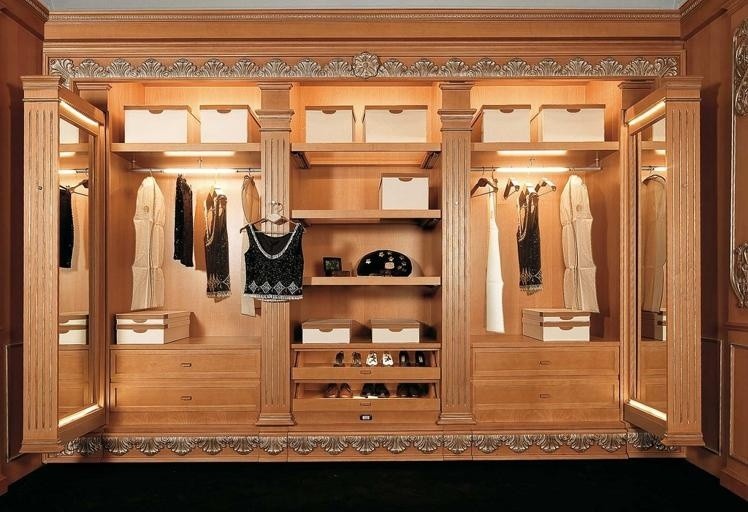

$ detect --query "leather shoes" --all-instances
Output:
[374,383,390,398]
[340,383,353,397]
[325,384,339,397]
[361,383,374,396]
[410,384,428,397]
[382,352,394,367]
[365,352,378,366]
[397,384,409,397]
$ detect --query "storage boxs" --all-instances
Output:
[363,104,428,143]
[197,105,261,144]
[304,104,358,143]
[535,104,608,143]
[115,330,190,344]
[641,309,666,322]
[58,329,90,347]
[640,324,668,342]
[60,313,90,325]
[472,103,532,142]
[521,308,592,324]
[122,105,201,145]
[378,172,432,213]
[302,319,352,344]
[116,310,192,328]
[369,319,421,344]
[523,321,592,343]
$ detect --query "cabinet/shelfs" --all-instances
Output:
[458,77,628,457]
[112,86,272,465]
[273,81,455,462]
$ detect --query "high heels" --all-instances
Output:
[350,352,362,367]
[398,351,411,366]
[334,352,345,367]
[416,350,425,366]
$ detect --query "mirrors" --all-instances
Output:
[17,77,108,455]
[624,79,705,448]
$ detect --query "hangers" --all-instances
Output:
[489,164,499,185]
[239,202,305,235]
[643,164,668,187]
[535,169,556,197]
[566,166,580,178]
[518,169,538,205]
[470,166,498,198]
[504,167,520,201]
[201,166,227,205]
[68,167,90,196]
[245,165,253,179]
[179,165,187,180]
[147,165,154,179]
